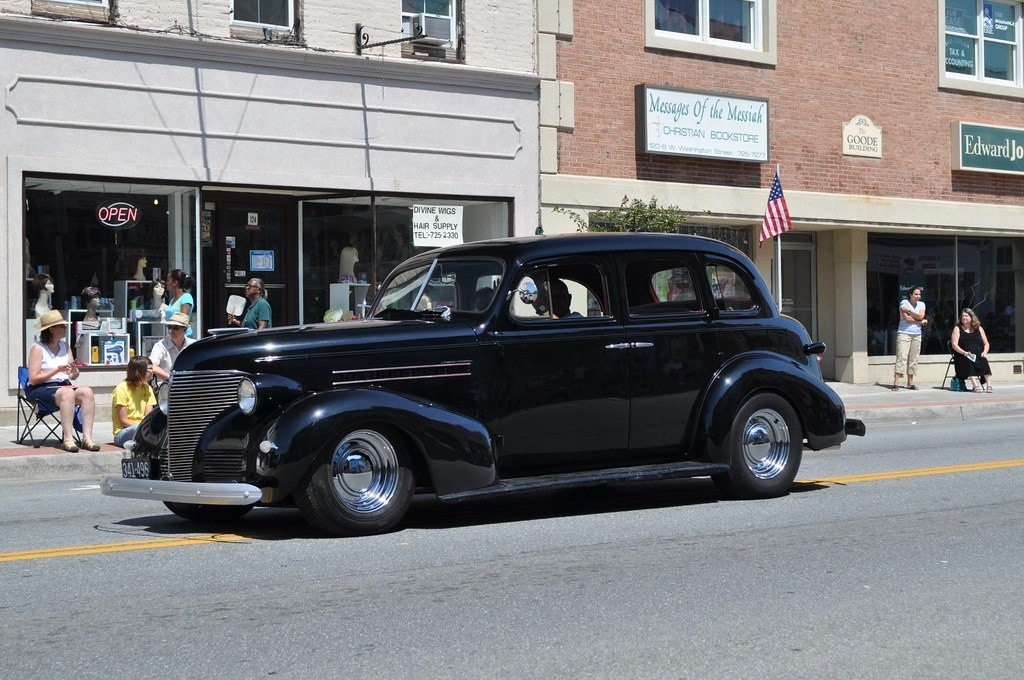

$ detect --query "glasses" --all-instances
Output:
[167,325,184,331]
[145,367,154,372]
[245,284,261,289]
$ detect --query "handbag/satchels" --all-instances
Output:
[951,376,967,392]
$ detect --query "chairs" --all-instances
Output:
[16,365,84,447]
[942,340,985,392]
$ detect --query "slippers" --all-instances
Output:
[907,385,919,390]
[892,385,898,391]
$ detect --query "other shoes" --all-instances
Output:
[987,386,993,393]
[61,440,79,452]
[82,437,100,451]
[974,386,981,392]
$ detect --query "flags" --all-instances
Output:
[758,171,792,248]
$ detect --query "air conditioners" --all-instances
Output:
[409,13,457,49]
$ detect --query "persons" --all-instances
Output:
[892,287,928,391]
[81,286,102,329]
[950,308,993,393]
[132,248,148,280]
[534,278,583,318]
[228,278,272,330]
[148,278,166,310]
[33,273,55,319]
[338,247,359,284]
[164,269,194,339]
[146,312,197,392]
[25,310,100,452]
[112,356,158,451]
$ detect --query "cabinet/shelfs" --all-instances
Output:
[65,279,169,365]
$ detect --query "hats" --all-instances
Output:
[34,310,73,332]
[161,312,190,327]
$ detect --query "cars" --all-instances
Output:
[100,232,866,537]
